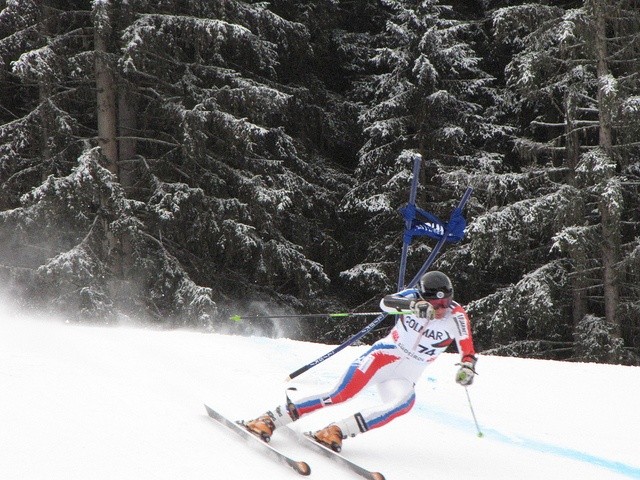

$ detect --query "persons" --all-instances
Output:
[236,271,477,453]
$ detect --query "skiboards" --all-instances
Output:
[278,419,385,480]
[205,404,311,477]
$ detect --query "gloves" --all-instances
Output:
[456,362,475,386]
[410,301,435,320]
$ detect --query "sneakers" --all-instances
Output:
[313,422,343,453]
[245,414,275,440]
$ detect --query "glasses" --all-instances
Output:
[425,295,454,310]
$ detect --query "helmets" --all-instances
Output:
[417,271,455,306]
